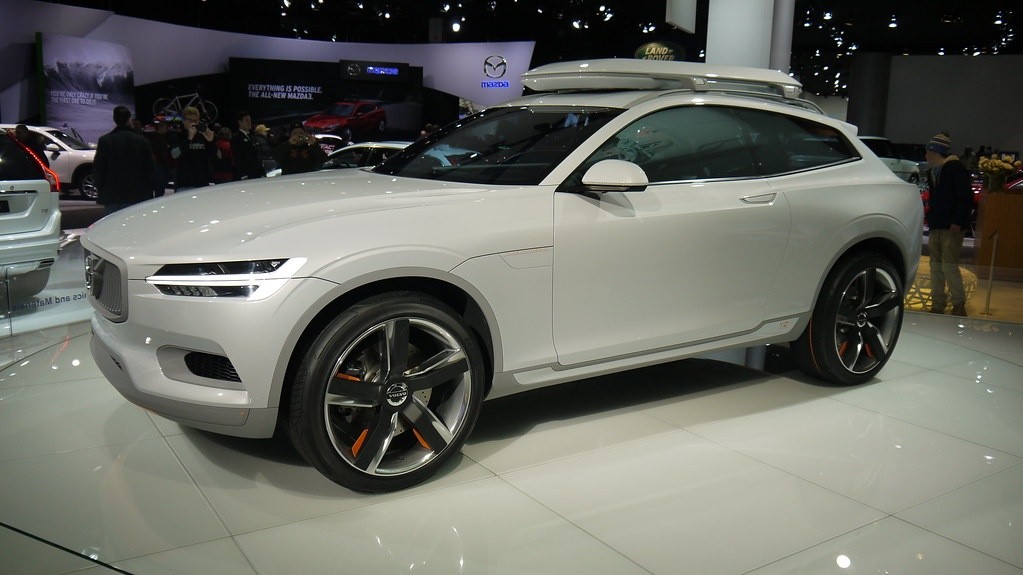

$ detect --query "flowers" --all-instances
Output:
[977,153,1022,174]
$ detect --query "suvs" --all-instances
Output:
[790,135,920,183]
[0,129,60,279]
[78,64,927,494]
[0,123,99,199]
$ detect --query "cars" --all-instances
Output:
[154,112,176,124]
[312,134,354,149]
[301,100,388,141]
[920,170,1023,231]
[265,140,489,179]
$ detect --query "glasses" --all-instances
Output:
[183,117,199,123]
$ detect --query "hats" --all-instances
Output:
[289,121,305,134]
[926,131,951,156]
[255,125,270,132]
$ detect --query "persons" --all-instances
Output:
[93,106,153,216]
[15,125,50,169]
[418,124,441,141]
[333,129,354,163]
[925,129,972,317]
[128,106,330,200]
[959,144,999,161]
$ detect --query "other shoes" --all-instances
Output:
[952,301,967,316]
[929,308,943,314]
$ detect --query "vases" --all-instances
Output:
[982,171,1004,191]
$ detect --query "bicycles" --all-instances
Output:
[153,86,218,125]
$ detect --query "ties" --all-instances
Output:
[247,133,256,146]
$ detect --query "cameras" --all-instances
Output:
[299,135,306,141]
[193,122,207,132]
